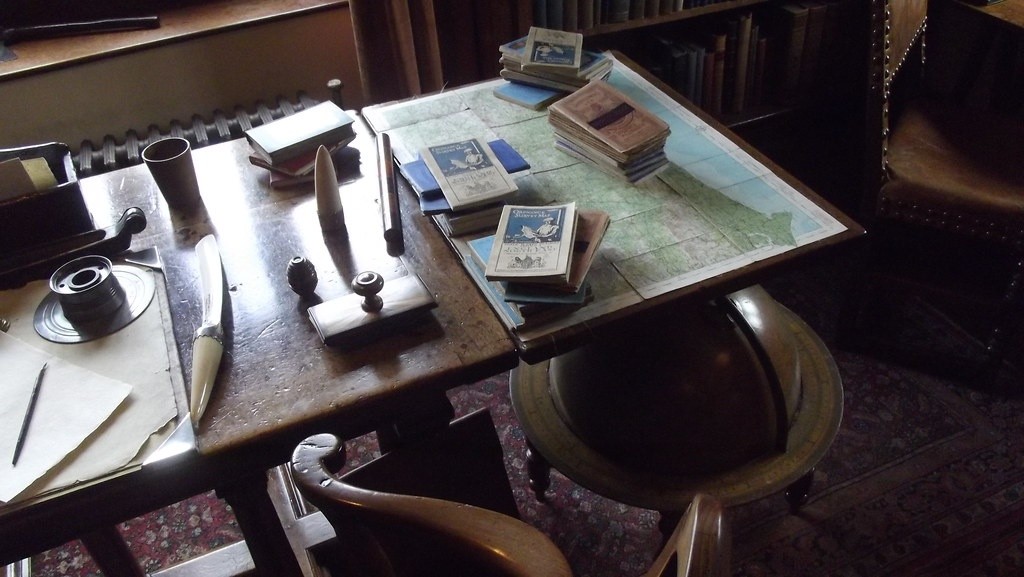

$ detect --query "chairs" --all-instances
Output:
[286,432,729,577]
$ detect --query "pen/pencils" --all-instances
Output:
[11,361,48,467]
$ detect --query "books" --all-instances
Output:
[533,0,848,115]
[402,29,669,331]
[245,100,357,187]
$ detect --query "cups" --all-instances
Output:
[141,137,200,209]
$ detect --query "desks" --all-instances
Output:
[0,48,871,564]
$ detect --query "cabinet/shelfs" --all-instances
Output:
[418,0,884,220]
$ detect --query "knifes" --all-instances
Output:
[191,234,223,424]
